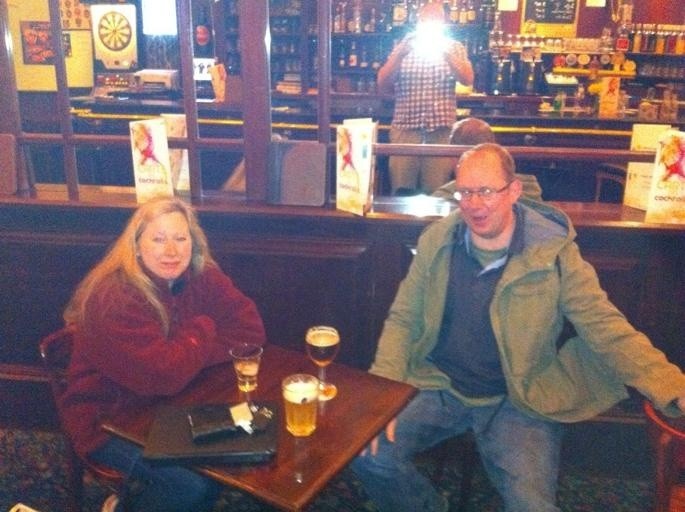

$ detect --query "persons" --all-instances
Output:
[346,144,685,512]
[428,118,543,198]
[59,196,266,512]
[377,2,475,195]
[21,27,42,64]
[35,29,56,64]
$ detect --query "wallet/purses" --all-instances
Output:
[187,405,237,441]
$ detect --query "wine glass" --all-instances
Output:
[306,323,342,403]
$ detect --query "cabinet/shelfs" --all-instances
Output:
[210,0,495,94]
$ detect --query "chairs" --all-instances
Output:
[39,324,130,512]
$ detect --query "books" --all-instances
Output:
[143,399,277,464]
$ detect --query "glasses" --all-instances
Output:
[460,181,510,199]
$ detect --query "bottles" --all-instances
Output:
[193,0,685,98]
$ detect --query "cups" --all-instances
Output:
[228,343,263,393]
[282,372,320,438]
